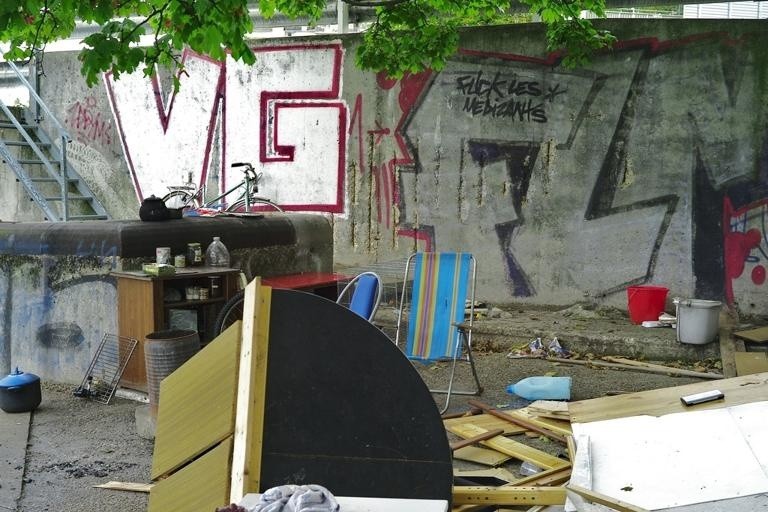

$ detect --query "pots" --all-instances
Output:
[167,205,191,220]
[0,365,42,413]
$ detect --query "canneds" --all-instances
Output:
[208,275,221,298]
[188,242,202,265]
[156,247,170,265]
[184,286,209,300]
[175,255,185,269]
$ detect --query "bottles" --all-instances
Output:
[138,194,168,221]
[186,286,209,299]
[207,276,222,297]
[187,242,202,266]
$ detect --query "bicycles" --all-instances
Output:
[160,161,283,213]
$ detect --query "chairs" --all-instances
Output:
[394,251,483,414]
[337,271,381,322]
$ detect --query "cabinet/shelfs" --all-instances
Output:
[112,266,241,396]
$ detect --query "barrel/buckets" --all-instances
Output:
[674,300,722,345]
[626,287,669,324]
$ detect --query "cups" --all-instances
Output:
[156,247,171,267]
[175,256,186,268]
